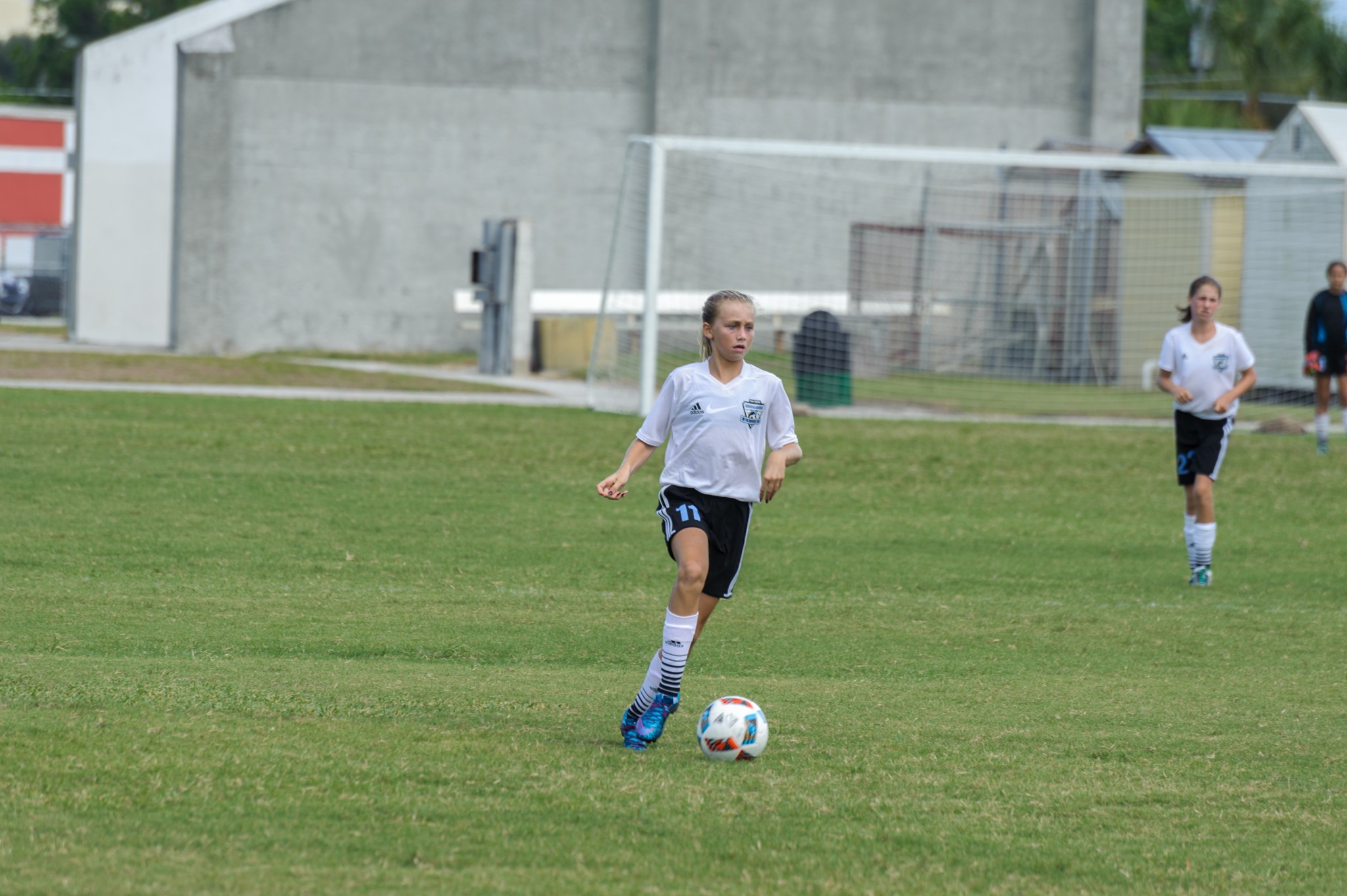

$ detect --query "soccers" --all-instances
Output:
[695,696,769,764]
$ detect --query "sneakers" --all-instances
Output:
[621,708,647,751]
[636,690,680,743]
[1189,566,1212,588]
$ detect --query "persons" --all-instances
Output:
[1157,276,1258,585]
[1304,262,1347,454]
[597,290,802,750]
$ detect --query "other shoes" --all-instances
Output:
[1316,439,1329,458]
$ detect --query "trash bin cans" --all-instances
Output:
[791,310,852,408]
